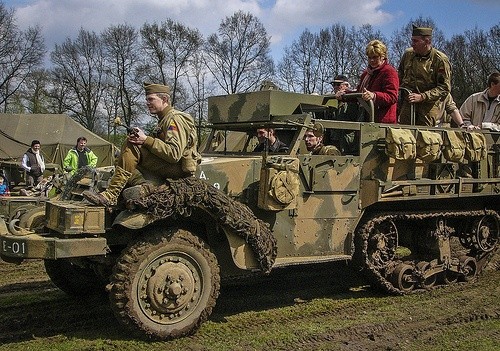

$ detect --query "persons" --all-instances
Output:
[435,94,466,128]
[63,137,98,175]
[304,129,323,154]
[336,40,399,122]
[254,121,289,152]
[21,140,45,185]
[459,72,500,131]
[330,75,354,118]
[83,81,197,214]
[0,170,10,196]
[396,24,451,126]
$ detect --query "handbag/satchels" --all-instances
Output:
[385,127,416,160]
[442,131,465,161]
[417,130,440,161]
[466,133,488,161]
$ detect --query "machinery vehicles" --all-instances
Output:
[1,88,500,341]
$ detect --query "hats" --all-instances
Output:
[330,75,349,83]
[412,24,432,36]
[143,81,170,95]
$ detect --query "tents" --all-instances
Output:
[0,113,114,184]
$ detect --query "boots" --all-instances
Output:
[121,170,153,199]
[83,165,133,211]
